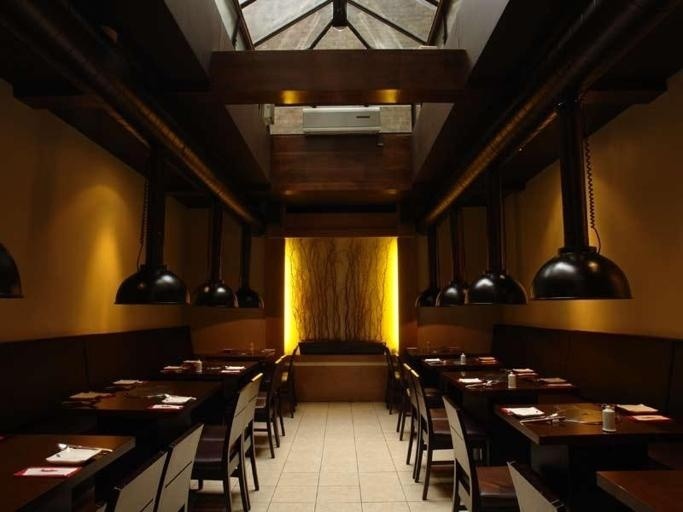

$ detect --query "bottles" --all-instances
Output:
[601,406,618,433]
[507,372,518,389]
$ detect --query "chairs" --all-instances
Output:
[394,353,436,440]
[380,343,444,441]
[72,450,166,510]
[505,459,571,512]
[97,424,203,510]
[191,382,254,511]
[403,363,452,479]
[410,370,488,503]
[442,395,548,511]
[237,343,301,459]
[194,369,263,508]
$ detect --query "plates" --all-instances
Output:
[458,376,481,384]
[509,407,544,417]
[616,403,659,415]
[46,446,103,465]
[162,395,192,405]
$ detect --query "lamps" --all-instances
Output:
[113,140,267,311]
[530,101,635,303]
[463,165,531,306]
[437,207,472,307]
[416,229,445,308]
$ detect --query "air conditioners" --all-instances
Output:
[302,107,382,133]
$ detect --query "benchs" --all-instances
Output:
[488,322,682,474]
[1,327,194,441]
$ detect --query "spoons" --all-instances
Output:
[58,443,114,453]
